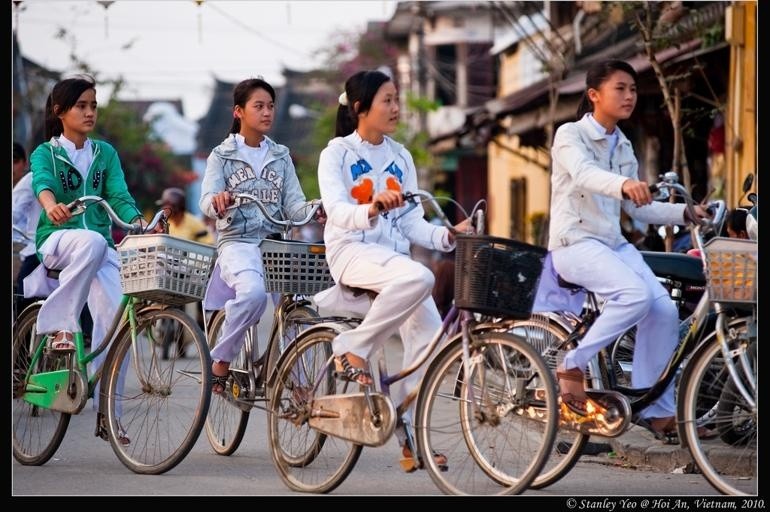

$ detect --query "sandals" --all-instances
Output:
[96,413,131,447]
[651,415,712,439]
[52,330,76,352]
[211,360,229,394]
[555,369,588,416]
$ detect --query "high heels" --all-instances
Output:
[334,352,374,387]
[399,451,447,471]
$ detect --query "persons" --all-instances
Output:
[13,143,45,315]
[29,72,163,445]
[155,185,213,246]
[546,59,711,443]
[197,73,326,403]
[310,71,475,473]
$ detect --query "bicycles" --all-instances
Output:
[266,189,559,496]
[175,190,338,468]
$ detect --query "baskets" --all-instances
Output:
[259,237,335,294]
[702,235,758,303]
[114,234,217,306]
[454,234,548,320]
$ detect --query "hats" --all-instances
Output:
[156,188,185,209]
